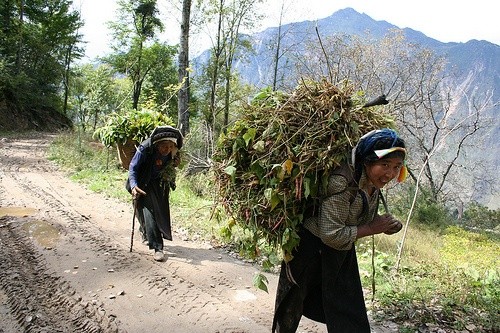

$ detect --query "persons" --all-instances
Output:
[126,126,184,261]
[270,128,407,333]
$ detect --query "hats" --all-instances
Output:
[149,127,183,146]
[356,128,406,162]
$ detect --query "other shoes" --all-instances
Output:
[153,251,165,261]
